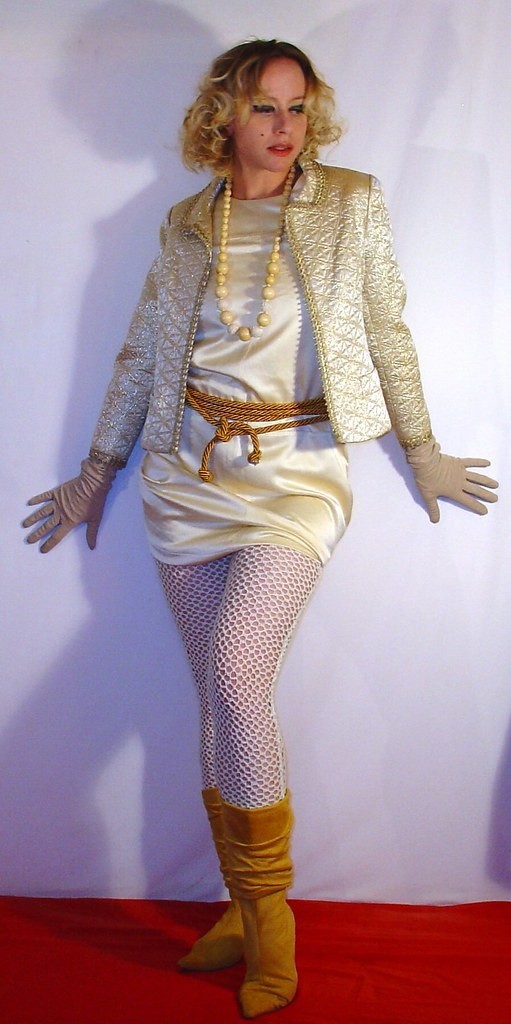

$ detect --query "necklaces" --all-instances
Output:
[215,168,295,341]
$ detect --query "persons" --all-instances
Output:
[24,38,501,1017]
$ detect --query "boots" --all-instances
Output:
[179,788,246,971]
[217,789,299,1018]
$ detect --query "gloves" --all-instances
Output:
[404,433,499,523]
[23,457,119,553]
